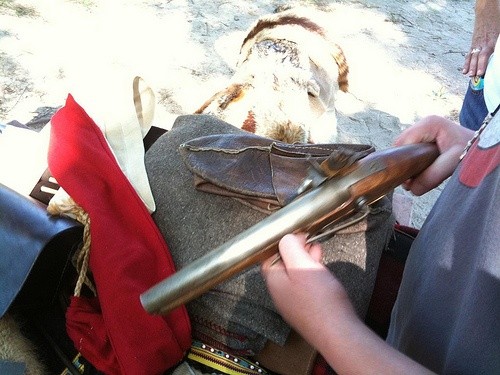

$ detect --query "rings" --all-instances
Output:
[470,48,481,54]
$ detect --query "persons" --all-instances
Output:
[262,103,500,375]
[458,0,500,132]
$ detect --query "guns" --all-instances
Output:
[139,141,441,316]
